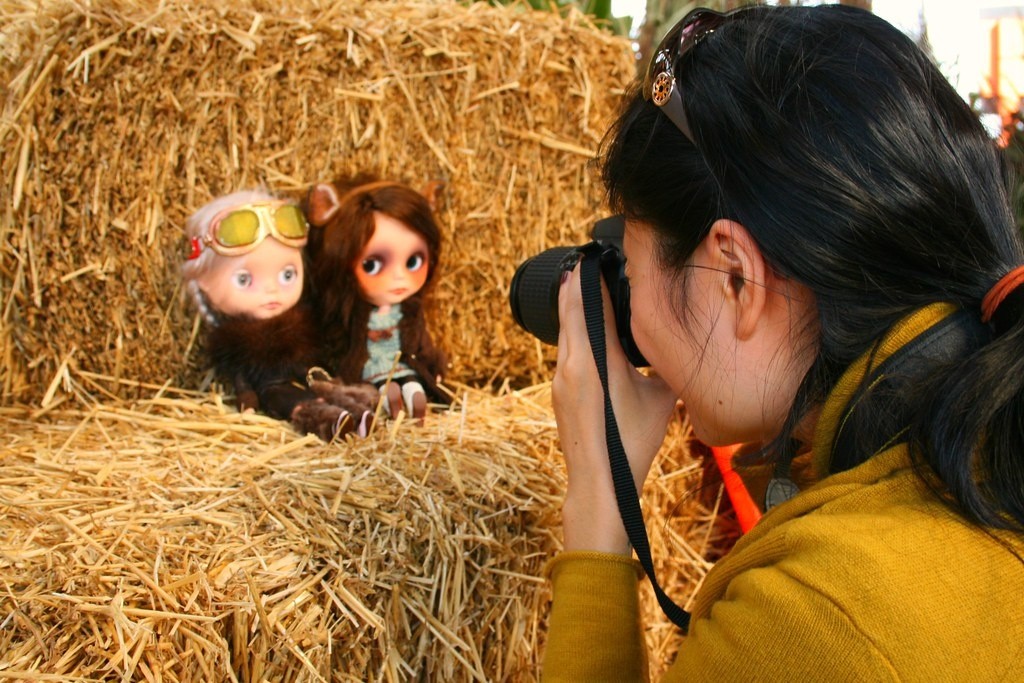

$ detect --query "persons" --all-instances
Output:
[175,169,446,443]
[540,0,1024,683]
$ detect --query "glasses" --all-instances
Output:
[642,4,776,150]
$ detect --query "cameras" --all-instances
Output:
[510,217,650,369]
